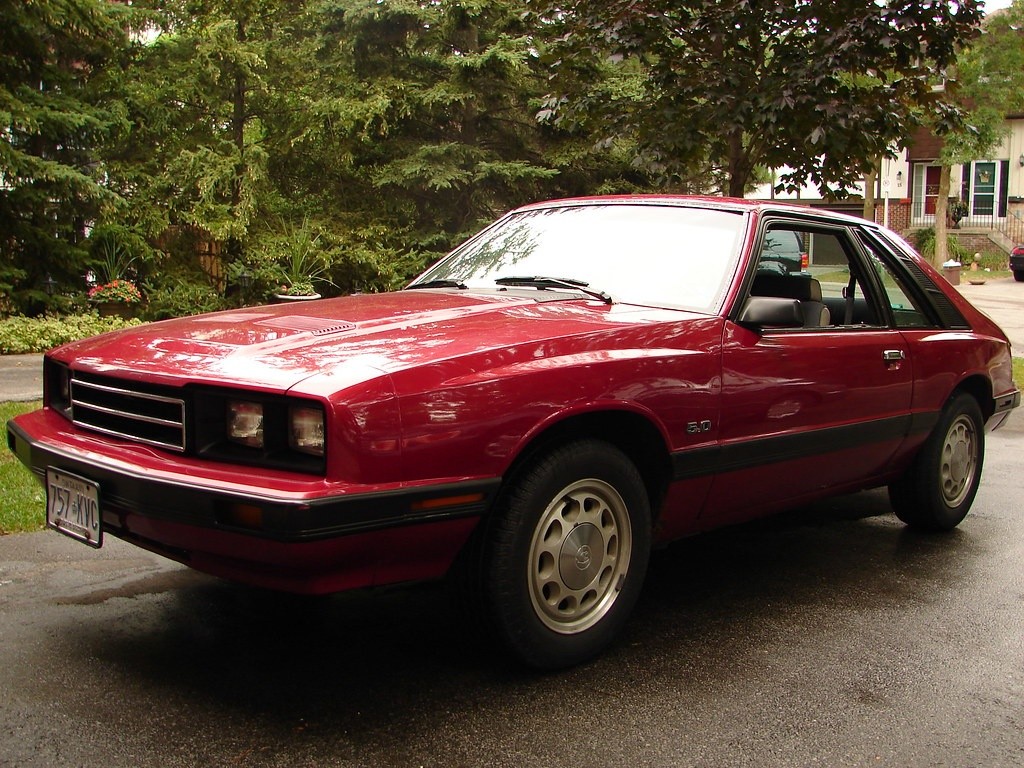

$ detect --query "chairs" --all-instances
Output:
[741,276,830,329]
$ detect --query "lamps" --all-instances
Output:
[896,171,902,187]
[1019,154,1024,167]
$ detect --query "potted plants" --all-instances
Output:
[951,191,963,229]
[94,231,142,321]
[265,211,341,305]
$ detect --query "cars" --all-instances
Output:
[1009,244,1024,283]
[4,194,1021,669]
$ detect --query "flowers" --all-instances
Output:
[278,283,317,296]
[948,201,969,222]
[88,279,143,307]
